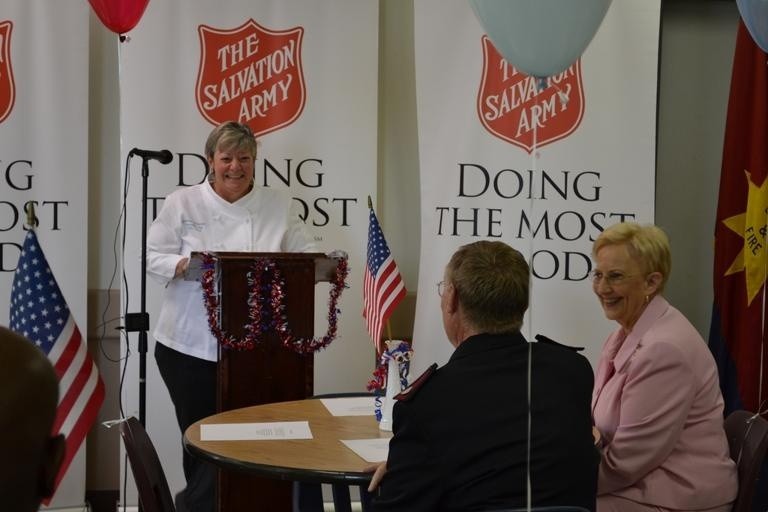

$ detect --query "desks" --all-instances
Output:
[182,396,393,512]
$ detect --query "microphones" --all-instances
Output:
[129,148,173,165]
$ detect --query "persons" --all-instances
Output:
[362,238,601,512]
[140,120,346,512]
[592,223,741,512]
[0,322,66,512]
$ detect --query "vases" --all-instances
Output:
[366,340,415,432]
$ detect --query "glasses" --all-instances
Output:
[589,269,649,283]
[438,280,445,296]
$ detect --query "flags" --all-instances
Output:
[703,1,767,512]
[359,194,409,359]
[7,201,106,507]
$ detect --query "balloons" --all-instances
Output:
[735,0,768,57]
[470,1,613,92]
[90,1,149,44]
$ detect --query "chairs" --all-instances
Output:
[120,416,176,512]
[725,410,768,512]
